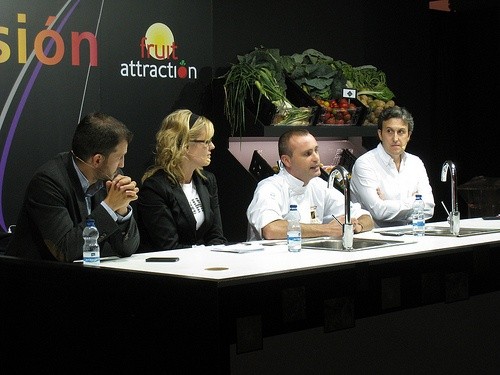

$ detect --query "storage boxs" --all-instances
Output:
[284,68,361,124]
[352,96,373,128]
[246,71,320,128]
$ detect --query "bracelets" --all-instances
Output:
[358,223,363,232]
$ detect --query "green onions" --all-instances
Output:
[220,61,292,136]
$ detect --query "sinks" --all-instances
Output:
[373,225,500,238]
[301,237,418,252]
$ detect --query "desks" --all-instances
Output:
[69,217,500,375]
[263,126,379,140]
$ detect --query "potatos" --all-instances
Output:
[359,95,395,125]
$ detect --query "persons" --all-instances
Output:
[4,112,140,263]
[350,106,435,228]
[246,128,374,241]
[136,109,227,252]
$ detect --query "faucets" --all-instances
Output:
[440,159,460,235]
[327,165,354,251]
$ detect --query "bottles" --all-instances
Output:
[412,194,425,237]
[286,204,302,253]
[82,220,100,266]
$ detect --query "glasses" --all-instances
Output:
[189,138,210,147]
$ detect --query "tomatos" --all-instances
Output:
[314,97,357,124]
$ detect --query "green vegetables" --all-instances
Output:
[235,45,395,100]
[273,108,312,126]
